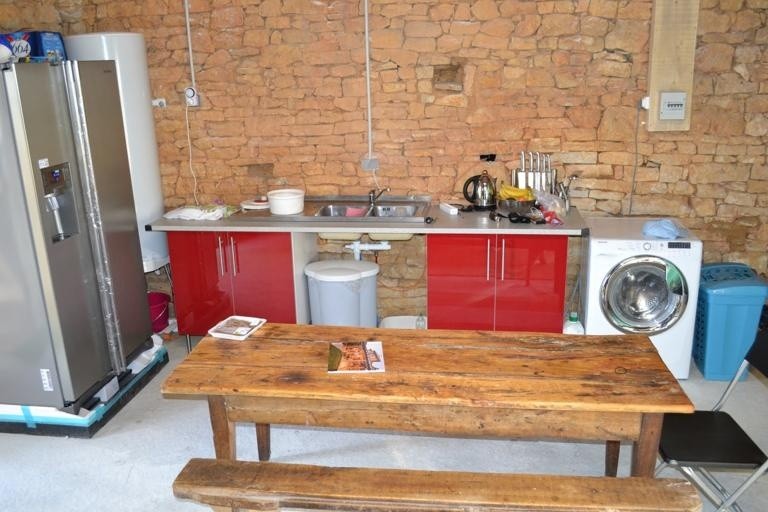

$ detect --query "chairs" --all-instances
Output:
[654,325,767,510]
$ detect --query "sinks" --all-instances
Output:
[368,200,430,223]
[314,203,370,222]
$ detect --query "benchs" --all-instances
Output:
[172,456,704,510]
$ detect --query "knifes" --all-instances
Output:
[519,150,555,192]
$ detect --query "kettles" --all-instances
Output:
[462,170,499,212]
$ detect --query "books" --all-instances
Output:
[327,340,385,374]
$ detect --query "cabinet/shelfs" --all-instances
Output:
[426,232,569,335]
[166,231,296,337]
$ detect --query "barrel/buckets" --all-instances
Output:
[148,291,171,331]
[563,310,585,335]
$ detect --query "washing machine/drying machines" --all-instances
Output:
[578,214,703,380]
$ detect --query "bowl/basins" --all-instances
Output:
[494,197,536,218]
[267,188,305,216]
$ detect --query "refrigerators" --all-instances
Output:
[0,59,153,413]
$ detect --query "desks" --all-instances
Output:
[161,321,696,475]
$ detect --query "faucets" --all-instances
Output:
[368,186,391,204]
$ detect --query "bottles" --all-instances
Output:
[563,313,584,335]
[417,313,425,328]
